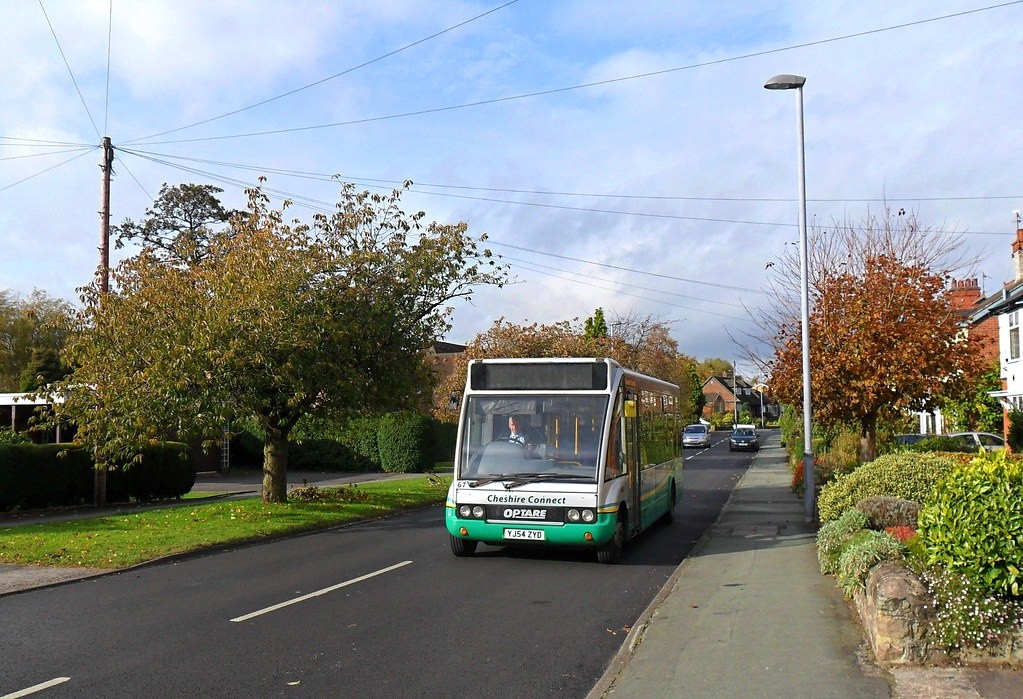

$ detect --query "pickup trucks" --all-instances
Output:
[683,424,711,448]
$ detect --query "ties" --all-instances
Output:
[514,437,518,443]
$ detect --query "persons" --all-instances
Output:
[495,415,537,452]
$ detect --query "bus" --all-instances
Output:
[446,357,683,563]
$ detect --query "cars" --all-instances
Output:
[729,428,760,452]
[947,432,1004,452]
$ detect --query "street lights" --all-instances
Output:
[609,321,621,359]
[764,74,814,522]
